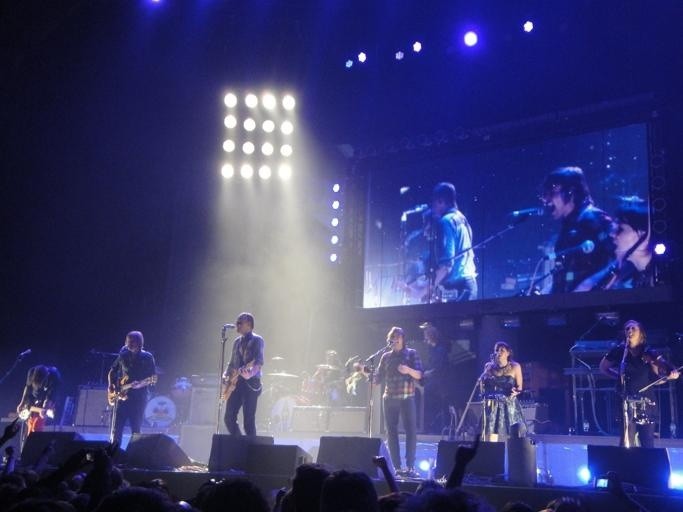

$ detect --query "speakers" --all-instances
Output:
[587,444,672,487]
[436,440,507,480]
[315,436,395,479]
[70,440,126,463]
[20,431,84,465]
[123,433,193,471]
[245,443,314,473]
[207,433,275,472]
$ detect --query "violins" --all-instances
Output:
[639,344,676,377]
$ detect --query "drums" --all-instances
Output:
[142,394,177,429]
[270,393,312,438]
[169,375,192,399]
[302,379,326,395]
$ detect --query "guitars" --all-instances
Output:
[16,401,56,422]
[106,372,159,406]
[221,358,255,400]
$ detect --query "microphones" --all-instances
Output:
[18,349,33,359]
[512,208,546,216]
[400,204,429,214]
[540,242,594,262]
[121,343,131,351]
[386,338,397,347]
[488,351,500,359]
[221,323,234,329]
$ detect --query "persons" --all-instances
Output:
[353,325,424,477]
[0,415,682,512]
[105,330,158,445]
[221,312,264,437]
[544,165,615,296]
[595,317,681,451]
[14,363,61,437]
[416,181,479,303]
[420,327,449,436]
[571,195,661,294]
[478,340,524,440]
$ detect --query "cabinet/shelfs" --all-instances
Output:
[571,356,680,438]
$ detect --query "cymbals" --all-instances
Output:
[266,373,298,378]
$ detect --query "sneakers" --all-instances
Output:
[394,470,408,477]
[408,471,419,478]
